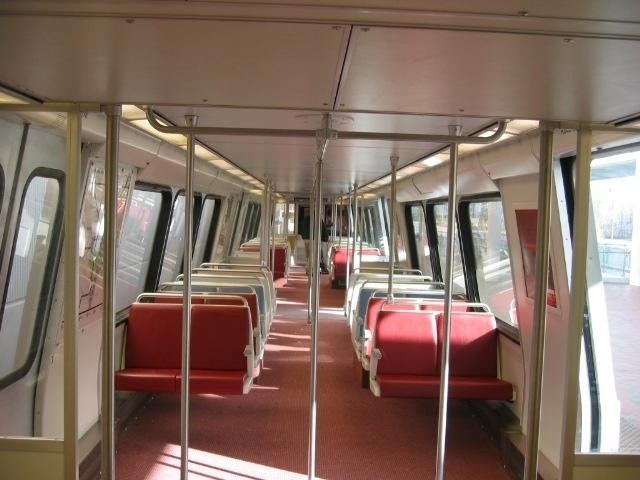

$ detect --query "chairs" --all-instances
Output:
[321,236,515,404]
[112,232,300,397]
